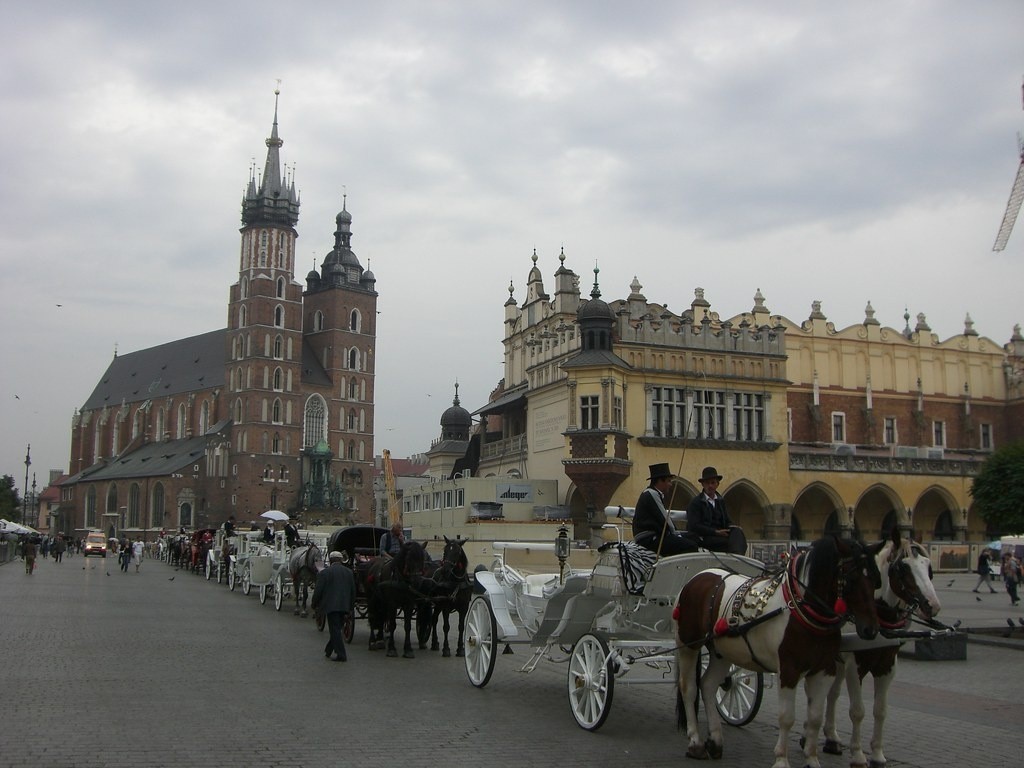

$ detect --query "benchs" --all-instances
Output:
[526,573,591,596]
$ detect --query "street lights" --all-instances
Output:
[22,445,31,526]
[31,472,38,528]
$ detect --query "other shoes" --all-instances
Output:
[991,591,997,593]
[973,590,980,593]
[1012,600,1020,606]
[331,655,346,662]
[325,646,333,657]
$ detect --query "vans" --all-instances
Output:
[84,532,107,558]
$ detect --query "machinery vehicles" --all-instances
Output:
[382,448,400,524]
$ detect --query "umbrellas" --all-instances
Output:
[109,538,118,542]
[260,510,290,521]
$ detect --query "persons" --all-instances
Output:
[1000,552,1024,606]
[194,527,199,540]
[17,530,87,563]
[284,515,304,550]
[250,521,262,532]
[263,520,276,544]
[202,530,212,541]
[225,516,238,538]
[632,463,698,557]
[180,527,185,534]
[687,467,748,556]
[312,551,357,662]
[380,522,406,563]
[110,532,151,575]
[973,548,998,594]
[21,539,37,574]
[160,528,167,539]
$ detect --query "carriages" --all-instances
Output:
[152,524,326,619]
[325,524,476,660]
[460,506,940,768]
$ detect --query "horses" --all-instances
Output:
[368,535,471,658]
[674,526,940,767]
[288,542,327,618]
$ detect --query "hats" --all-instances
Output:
[698,467,722,483]
[646,462,675,480]
[289,515,298,520]
[329,551,343,558]
